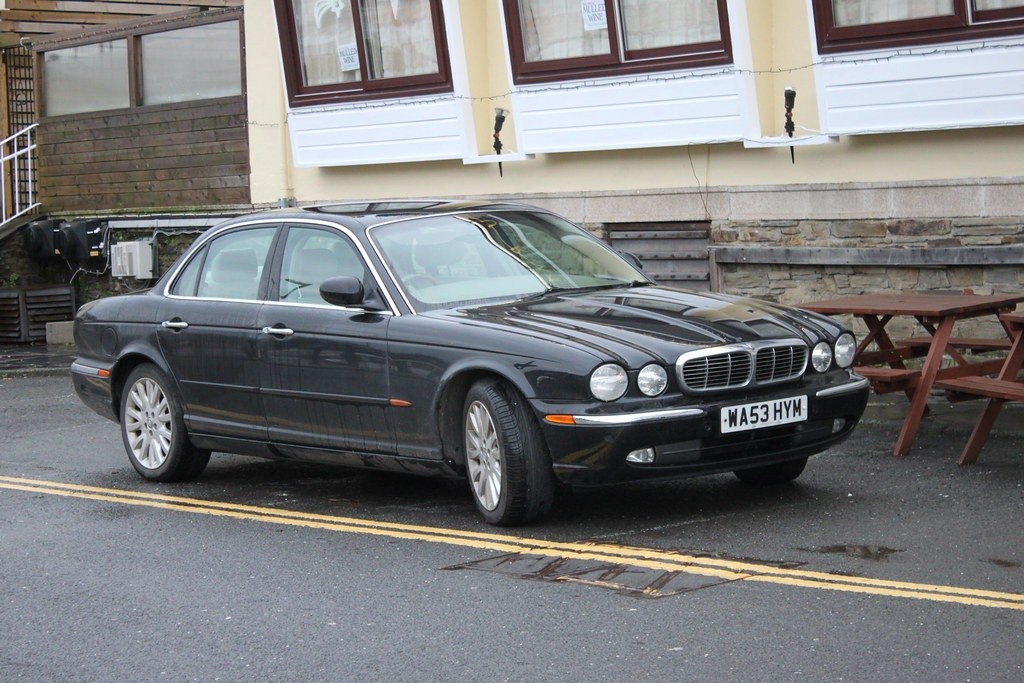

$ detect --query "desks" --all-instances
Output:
[955,312,1024,466]
[791,292,1024,457]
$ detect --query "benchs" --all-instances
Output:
[852,366,923,396]
[895,337,1013,361]
[934,375,1024,404]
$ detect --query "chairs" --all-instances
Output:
[295,248,339,302]
[206,248,260,299]
[328,240,361,277]
[413,230,467,285]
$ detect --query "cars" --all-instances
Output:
[70,197,871,525]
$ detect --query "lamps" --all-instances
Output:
[493,107,511,134]
[784,86,796,112]
